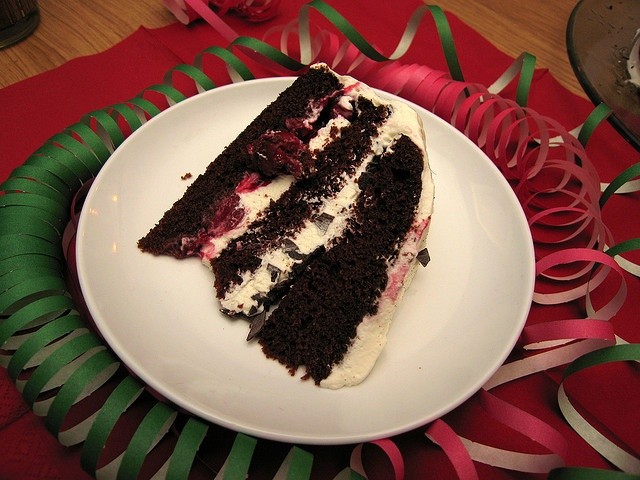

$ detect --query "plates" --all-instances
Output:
[75,73,537,446]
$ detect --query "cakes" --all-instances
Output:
[137,60,440,390]
